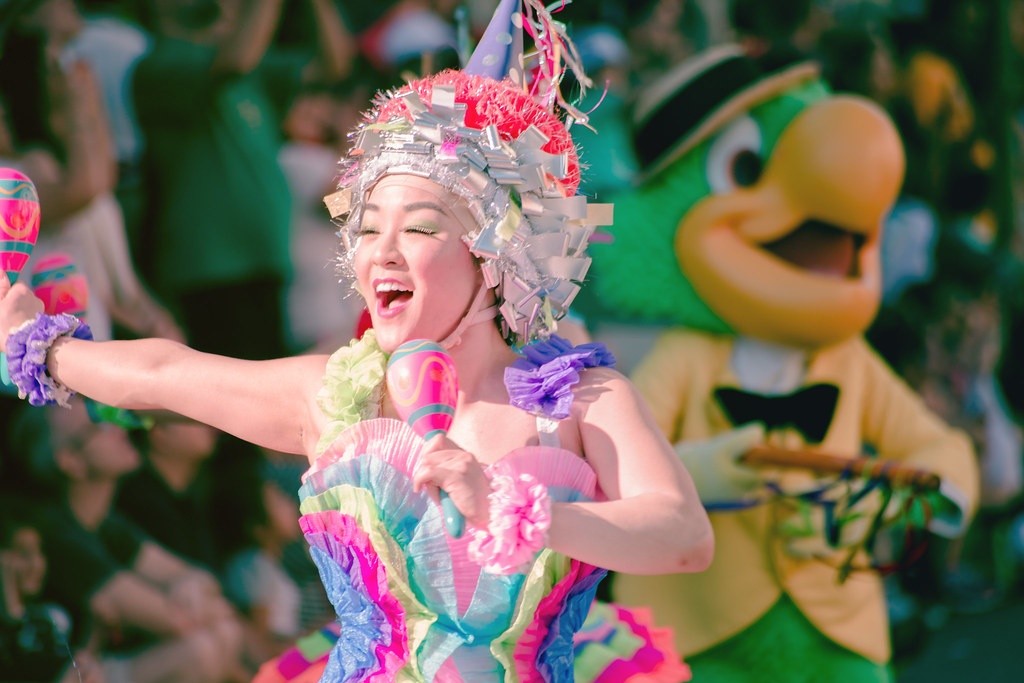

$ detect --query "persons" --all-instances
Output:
[0,0,714,683]
[559,1,1024,645]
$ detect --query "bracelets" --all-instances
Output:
[2,315,92,409]
[466,468,548,576]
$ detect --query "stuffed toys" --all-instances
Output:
[590,43,979,682]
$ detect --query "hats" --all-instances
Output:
[337,69,613,347]
